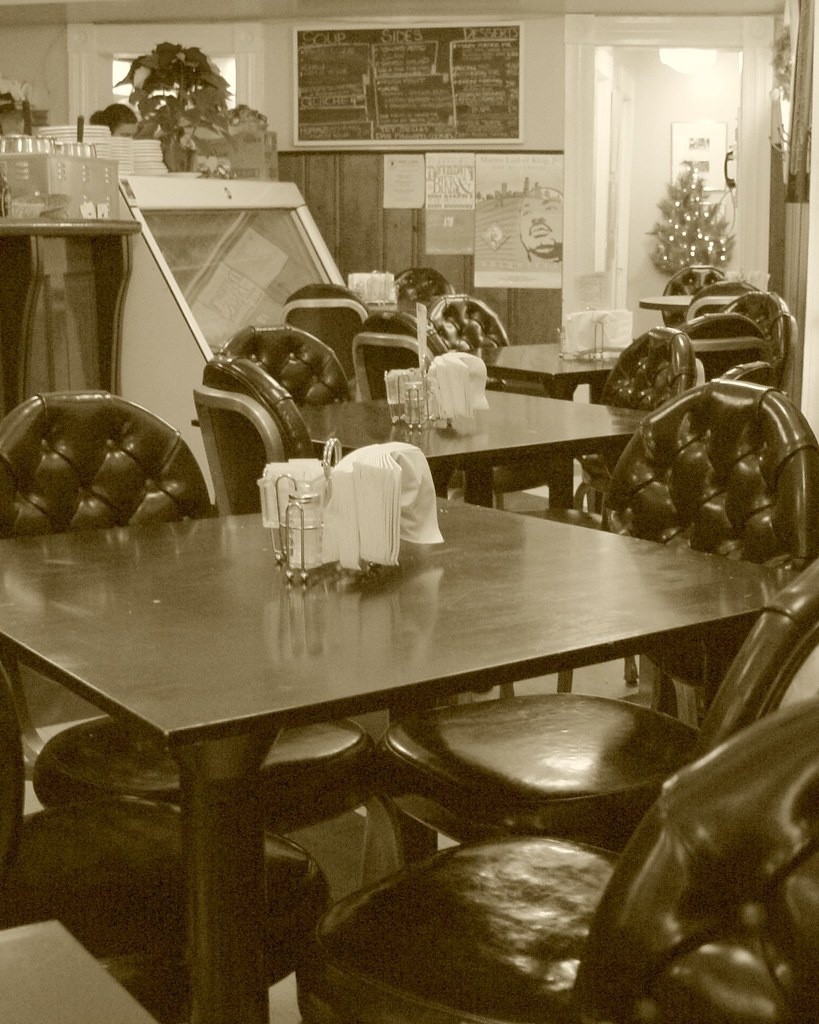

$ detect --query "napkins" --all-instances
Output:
[253,439,443,570]
[563,308,632,358]
[381,350,488,435]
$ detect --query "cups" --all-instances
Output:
[1,133,97,217]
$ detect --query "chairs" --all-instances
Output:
[195,261,459,515]
[0,668,325,1024]
[603,377,819,725]
[0,392,406,883]
[607,265,805,404]
[294,562,819,1023]
[433,295,512,392]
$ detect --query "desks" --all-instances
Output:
[292,389,652,513]
[639,295,696,312]
[475,343,627,400]
[0,499,785,1024]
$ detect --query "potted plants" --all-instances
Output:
[114,44,232,173]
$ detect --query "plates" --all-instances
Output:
[36,126,203,178]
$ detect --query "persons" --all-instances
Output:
[89,103,139,139]
